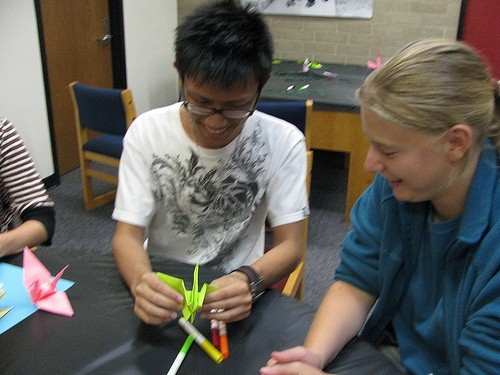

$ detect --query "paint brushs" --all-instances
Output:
[210,318,229,360]
[178,317,223,364]
[300,83,309,91]
[286,85,295,91]
[166,335,194,375]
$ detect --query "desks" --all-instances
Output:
[256,59,382,226]
[0,247,405,374]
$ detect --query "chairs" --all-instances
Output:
[69,80,139,210]
[252,100,313,301]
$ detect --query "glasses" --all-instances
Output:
[182,76,261,119]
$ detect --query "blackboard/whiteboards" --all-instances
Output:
[240,0,373,21]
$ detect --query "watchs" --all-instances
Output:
[229,266,265,303]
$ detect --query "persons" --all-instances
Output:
[0,117,56,262]
[113,0,311,329]
[259,37,500,375]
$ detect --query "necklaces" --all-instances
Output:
[188,117,199,145]
